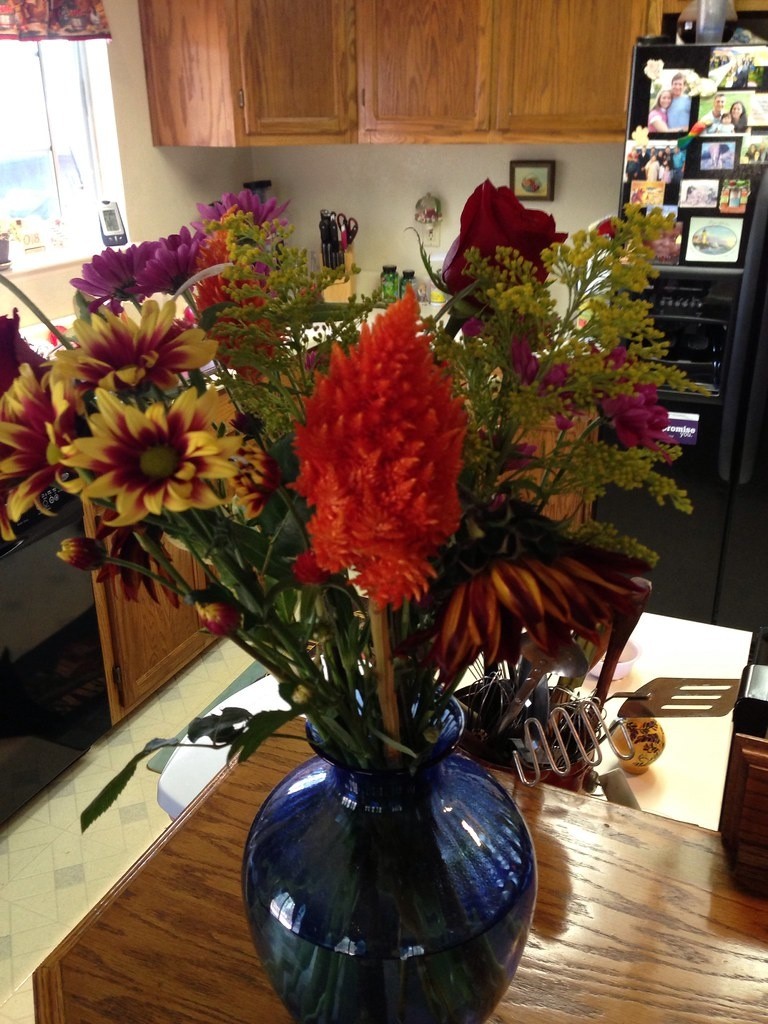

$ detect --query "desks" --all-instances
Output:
[153,610,753,830]
[32,721,767,1024]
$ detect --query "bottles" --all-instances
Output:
[400,270,417,300]
[380,265,399,302]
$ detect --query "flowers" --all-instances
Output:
[0,178,719,830]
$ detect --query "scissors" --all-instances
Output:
[337,213,360,248]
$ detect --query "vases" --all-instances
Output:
[240,687,539,1024]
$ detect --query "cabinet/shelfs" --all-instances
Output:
[137,0,358,148]
[646,0,768,38]
[355,0,650,144]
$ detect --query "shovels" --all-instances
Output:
[603,676,742,718]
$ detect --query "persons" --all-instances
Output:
[697,93,748,134]
[625,145,686,183]
[745,143,768,164]
[649,73,692,133]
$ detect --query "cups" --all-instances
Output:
[729,188,741,208]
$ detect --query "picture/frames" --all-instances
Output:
[509,160,556,203]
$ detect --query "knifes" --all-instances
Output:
[319,210,341,270]
